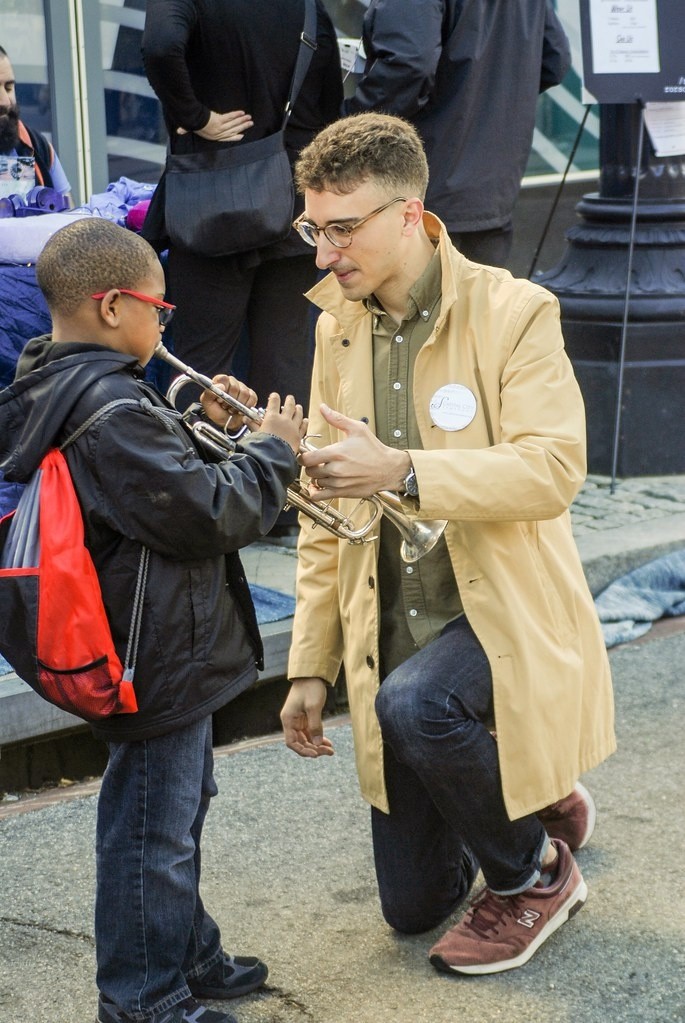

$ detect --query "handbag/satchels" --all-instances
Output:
[164,131,295,258]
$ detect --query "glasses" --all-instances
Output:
[91,290,176,325]
[292,196,408,249]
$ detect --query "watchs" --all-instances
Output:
[399,461,419,502]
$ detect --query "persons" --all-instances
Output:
[140,0,345,409]
[0,217,311,1022]
[0,47,74,216]
[342,0,570,268]
[281,112,618,976]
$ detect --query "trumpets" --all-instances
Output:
[154,343,449,564]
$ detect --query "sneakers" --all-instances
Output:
[96,991,236,1023]
[429,838,588,974]
[534,781,596,852]
[185,951,268,998]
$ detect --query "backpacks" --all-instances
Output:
[0,399,195,722]
